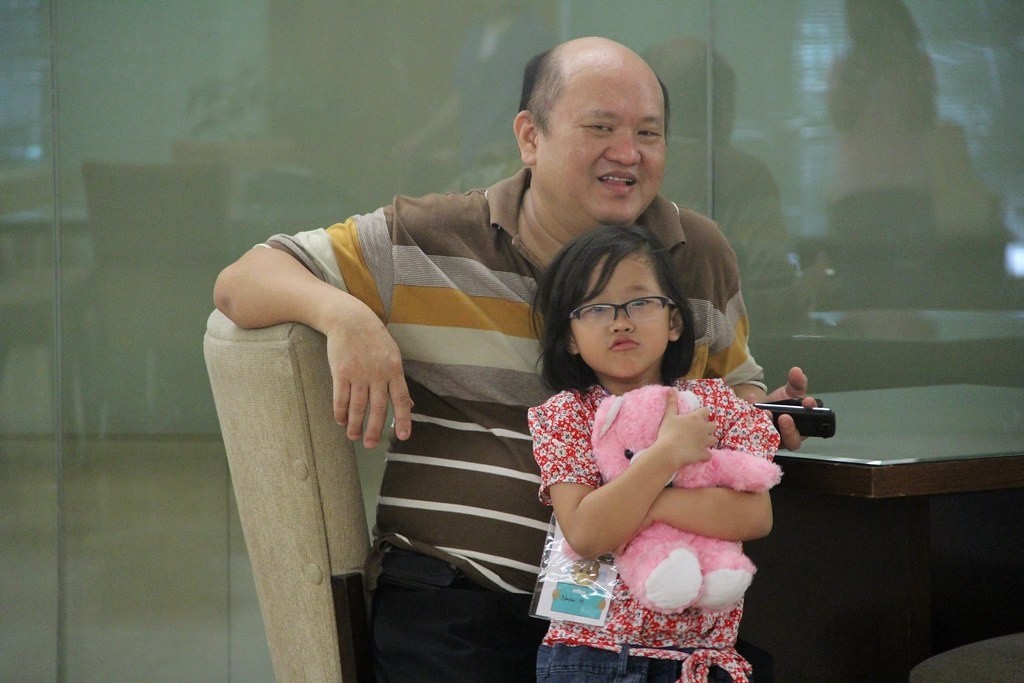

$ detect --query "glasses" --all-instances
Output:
[567,295,676,328]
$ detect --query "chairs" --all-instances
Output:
[203,306,374,683]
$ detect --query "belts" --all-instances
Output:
[381,546,490,591]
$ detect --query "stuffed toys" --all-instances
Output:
[591,384,783,615]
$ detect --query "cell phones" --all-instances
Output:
[753,404,835,439]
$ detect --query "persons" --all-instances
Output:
[213,36,819,683]
[527,227,779,682]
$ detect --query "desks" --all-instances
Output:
[735,383,1024,683]
